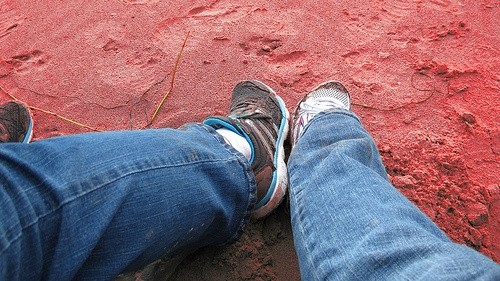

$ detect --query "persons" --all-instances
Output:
[288,80,500,281]
[0,80,290,281]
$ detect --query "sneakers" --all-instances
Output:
[203,80,290,219]
[0,100,33,144]
[287,80,350,208]
